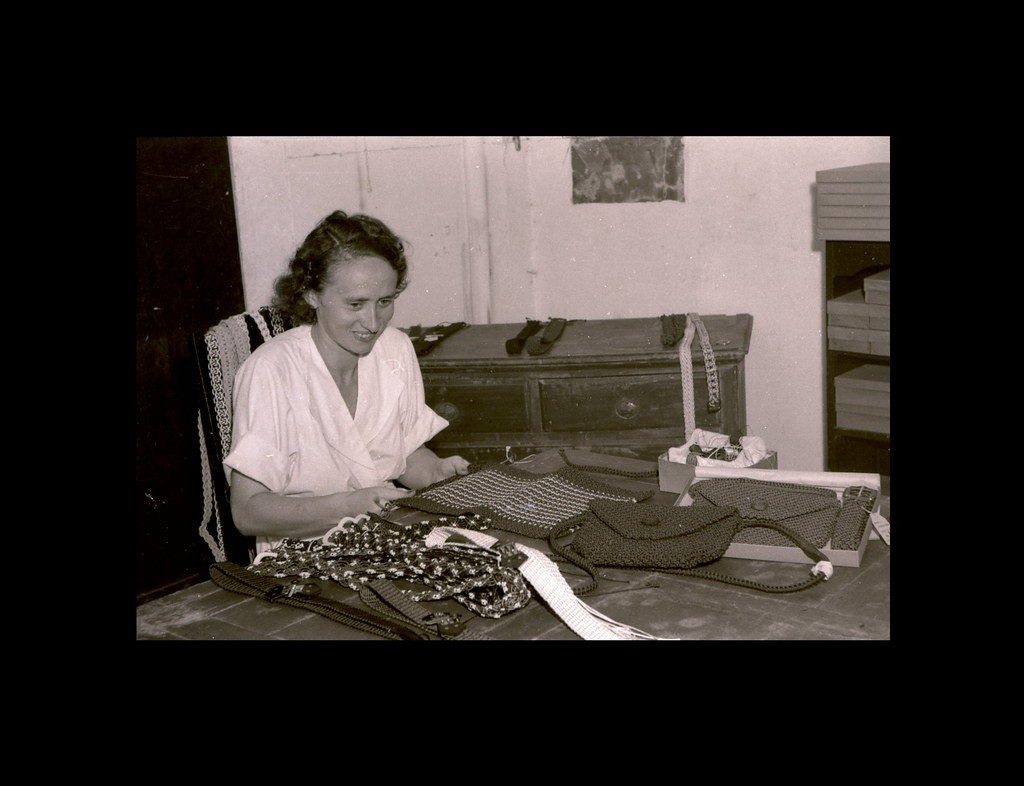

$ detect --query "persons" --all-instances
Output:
[222,209,472,558]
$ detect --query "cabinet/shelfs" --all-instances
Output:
[810,163,891,472]
[397,313,760,466]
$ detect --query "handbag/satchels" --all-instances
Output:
[571,498,739,568]
[692,477,841,550]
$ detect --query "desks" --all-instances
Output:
[136,448,890,640]
